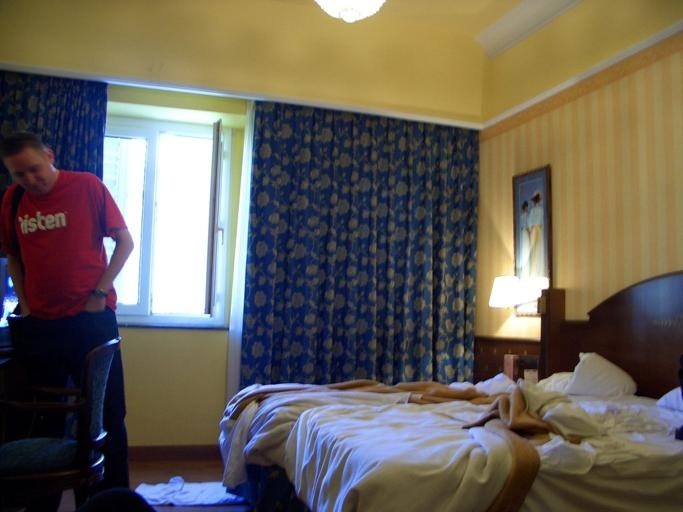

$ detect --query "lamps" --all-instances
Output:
[488,275,548,307]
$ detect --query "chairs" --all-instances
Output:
[0,338,120,511]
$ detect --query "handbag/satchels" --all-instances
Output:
[7,185,26,349]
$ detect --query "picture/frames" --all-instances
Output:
[510,162,551,316]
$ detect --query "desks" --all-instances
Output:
[0,348,21,506]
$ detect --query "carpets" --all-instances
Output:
[150,506,248,512]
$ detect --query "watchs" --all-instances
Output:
[92,289,107,298]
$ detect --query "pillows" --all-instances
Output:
[535,353,636,395]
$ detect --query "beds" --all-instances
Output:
[217,271,683,512]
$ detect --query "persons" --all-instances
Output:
[520,189,547,279]
[1,128,134,512]
